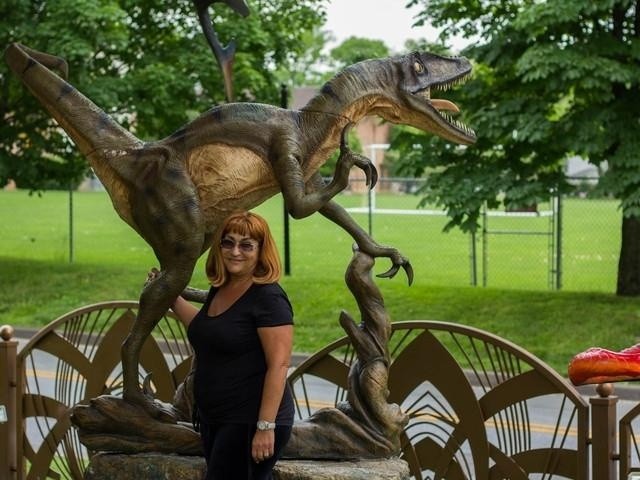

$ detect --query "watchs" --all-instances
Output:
[256,420,276,430]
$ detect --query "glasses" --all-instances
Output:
[222,239,259,252]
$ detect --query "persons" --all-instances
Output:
[143,211,296,480]
